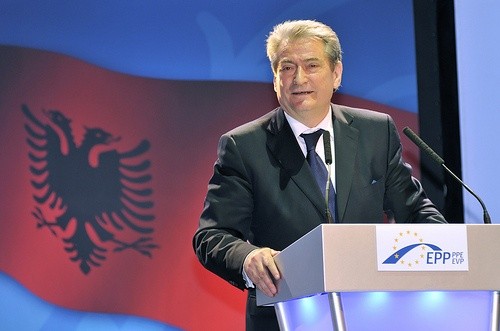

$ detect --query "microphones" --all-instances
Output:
[402,126,491,224]
[322,130,334,223]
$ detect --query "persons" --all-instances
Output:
[189,18,452,331]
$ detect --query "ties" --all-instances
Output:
[301,129,337,222]
